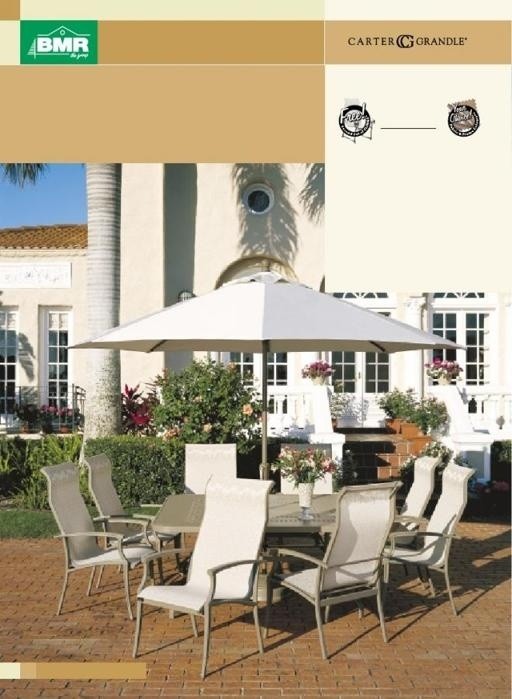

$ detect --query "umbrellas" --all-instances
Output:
[66,261,470,479]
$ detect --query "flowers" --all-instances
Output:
[300,359,335,378]
[35,403,56,423]
[54,405,82,425]
[17,401,38,421]
[422,357,464,379]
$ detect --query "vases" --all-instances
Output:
[41,424,55,433]
[436,376,452,385]
[59,425,72,434]
[20,422,33,433]
[310,375,325,386]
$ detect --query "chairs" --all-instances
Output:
[40,429,477,681]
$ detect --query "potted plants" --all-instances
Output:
[396,398,448,440]
[375,388,420,434]
